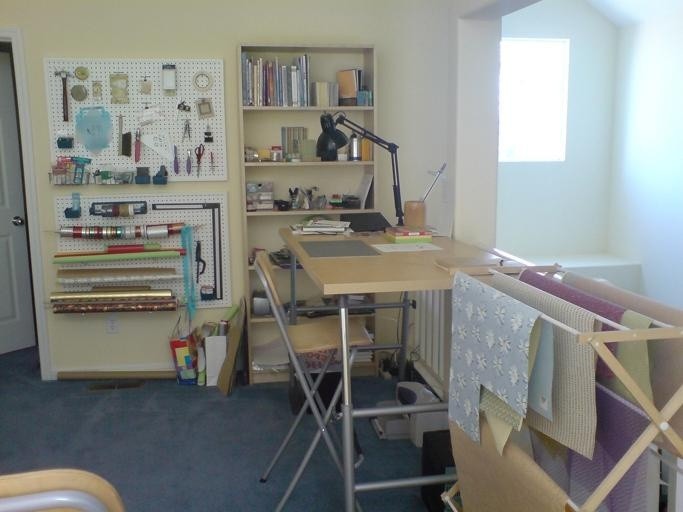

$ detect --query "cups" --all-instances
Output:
[402,199,427,231]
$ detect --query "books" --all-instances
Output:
[279,124,306,161]
[279,293,375,319]
[247,201,274,211]
[240,51,374,106]
[359,171,373,208]
[348,328,376,364]
[247,192,274,202]
[290,217,352,237]
[382,224,434,244]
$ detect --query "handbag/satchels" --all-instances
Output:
[169,322,228,387]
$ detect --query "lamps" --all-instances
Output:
[314,111,404,225]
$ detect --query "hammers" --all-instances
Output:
[55,71,75,122]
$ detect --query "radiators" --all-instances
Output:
[414,290,449,403]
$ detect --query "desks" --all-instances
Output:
[279,226,530,509]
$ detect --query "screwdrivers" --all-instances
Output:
[196,240,201,283]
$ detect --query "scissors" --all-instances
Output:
[195,144,205,178]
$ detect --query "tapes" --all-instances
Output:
[193,71,213,92]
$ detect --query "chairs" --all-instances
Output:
[250,249,373,512]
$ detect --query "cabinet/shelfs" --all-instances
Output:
[236,44,380,385]
